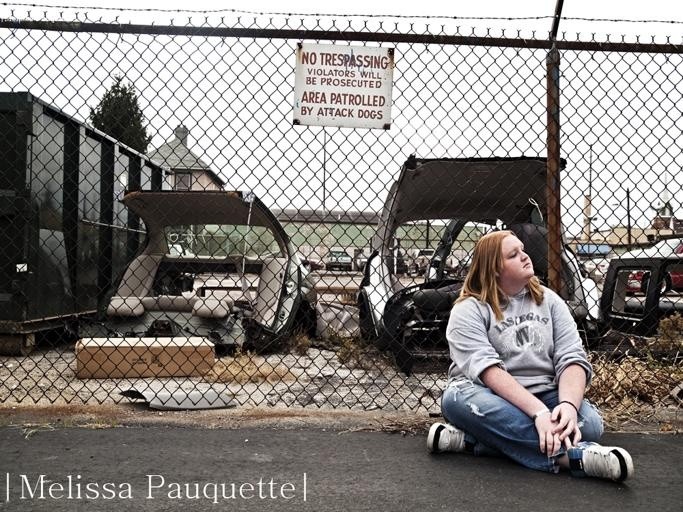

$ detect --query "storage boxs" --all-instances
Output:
[72,336,213,378]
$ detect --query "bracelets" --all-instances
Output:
[528,400,580,420]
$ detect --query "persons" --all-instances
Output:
[424,230,637,482]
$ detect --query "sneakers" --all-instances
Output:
[427,422,466,454]
[568,440,634,484]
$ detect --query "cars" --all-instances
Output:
[578,232,683,299]
[323,246,440,277]
